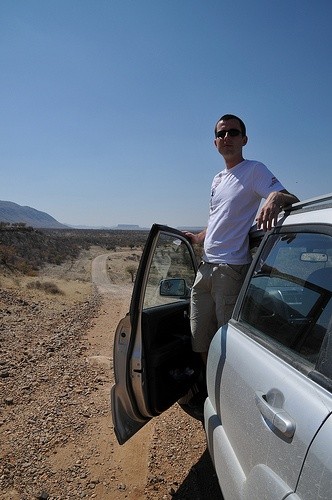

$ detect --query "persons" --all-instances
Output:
[180,114,303,363]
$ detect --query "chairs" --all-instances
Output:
[291,267,331,357]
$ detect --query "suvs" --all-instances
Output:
[111,191,332,500]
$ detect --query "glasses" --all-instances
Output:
[214,127,242,138]
